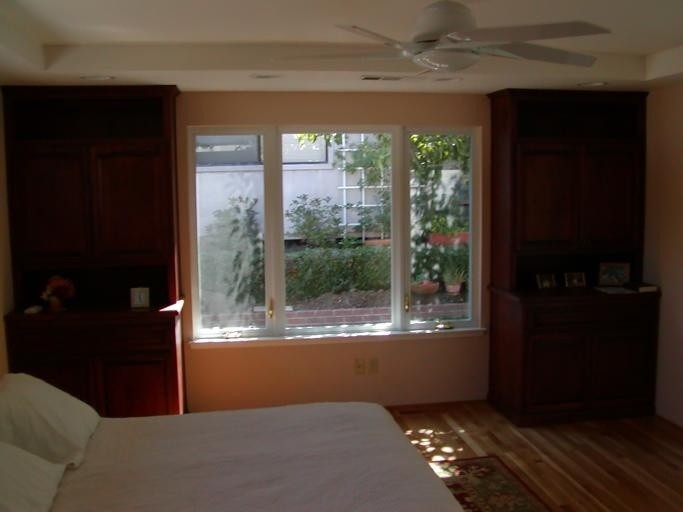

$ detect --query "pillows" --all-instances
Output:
[0,375,99,512]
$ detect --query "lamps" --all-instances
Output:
[412,45,481,76]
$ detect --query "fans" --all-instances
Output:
[331,1,609,80]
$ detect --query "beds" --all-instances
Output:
[39,401,461,512]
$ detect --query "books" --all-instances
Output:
[625,280,659,294]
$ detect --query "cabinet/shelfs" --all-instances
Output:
[481,88,661,432]
[1,84,184,420]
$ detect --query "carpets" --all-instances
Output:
[427,452,550,511]
